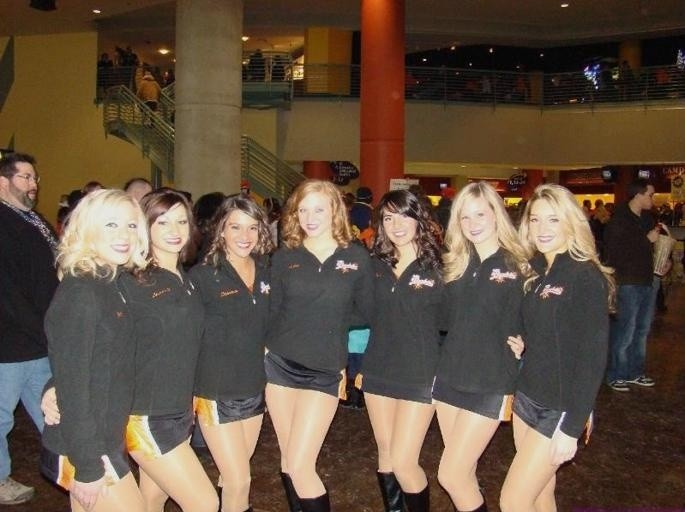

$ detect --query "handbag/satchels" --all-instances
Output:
[652,222,673,276]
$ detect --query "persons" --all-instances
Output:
[432,182,529,512]
[40,185,221,511]
[0,43,685,328]
[263,179,377,512]
[40,189,151,510]
[190,193,268,512]
[605,181,678,392]
[352,191,524,511]
[489,183,618,512]
[0,152,65,505]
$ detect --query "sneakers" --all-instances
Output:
[632,377,656,389]
[610,382,632,394]
[1,474,37,508]
